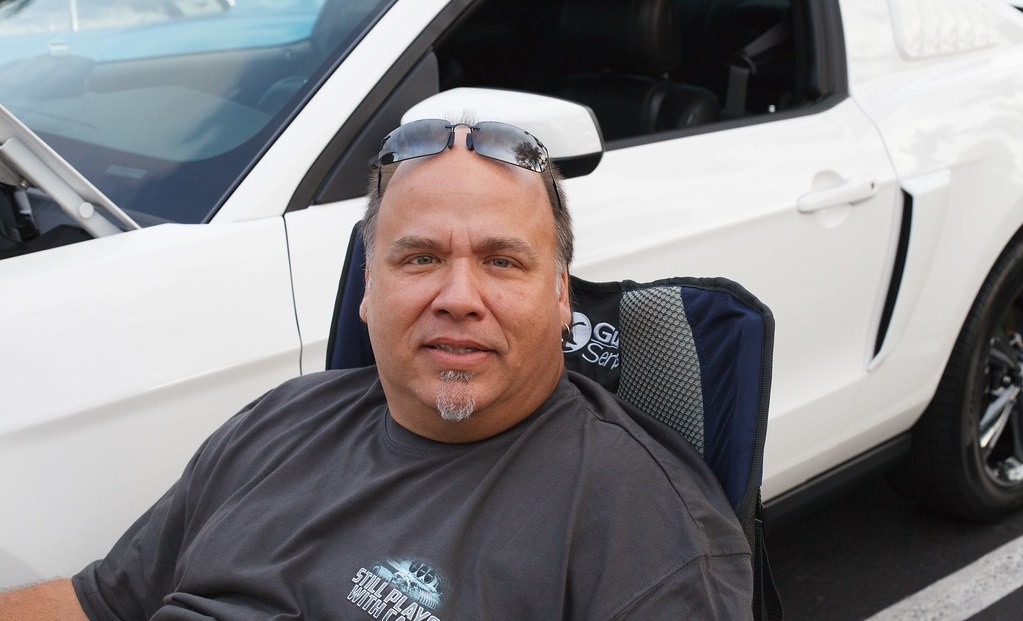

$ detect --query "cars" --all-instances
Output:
[0,0,1023,593]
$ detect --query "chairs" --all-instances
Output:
[324,220,786,621]
[523,0,717,148]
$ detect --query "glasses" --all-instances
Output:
[377,119,563,211]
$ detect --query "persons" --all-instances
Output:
[0,120,754,621]
